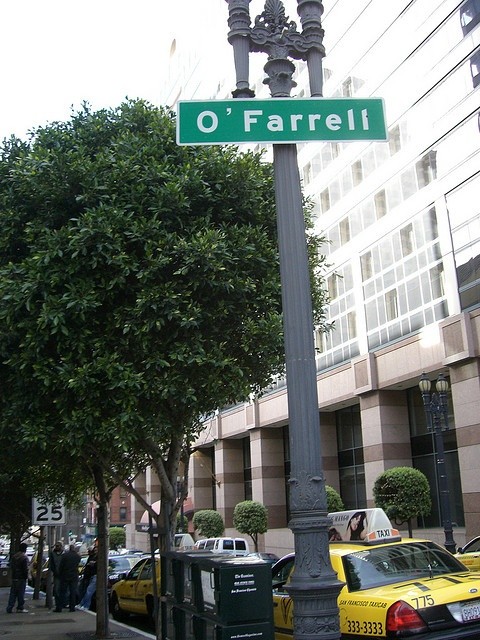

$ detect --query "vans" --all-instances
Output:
[196,537,251,561]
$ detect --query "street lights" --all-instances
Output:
[418,370,458,554]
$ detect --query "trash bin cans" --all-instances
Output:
[0,562,13,586]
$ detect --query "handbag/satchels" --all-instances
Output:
[85,562,95,577]
[79,578,89,590]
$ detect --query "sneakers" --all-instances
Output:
[79,606,88,612]
[74,604,81,609]
[18,609,28,613]
[70,609,75,612]
[53,609,61,612]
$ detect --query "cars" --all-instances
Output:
[77,556,91,578]
[77,553,143,603]
[27,556,90,597]
[272,507,480,639]
[246,552,281,565]
[25,549,36,562]
[452,534,480,578]
[109,533,198,623]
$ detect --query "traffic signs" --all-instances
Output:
[176,97,389,146]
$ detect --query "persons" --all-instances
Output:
[48,542,66,607]
[53,543,81,612]
[75,537,98,613]
[5,543,29,614]
[329,511,368,542]
